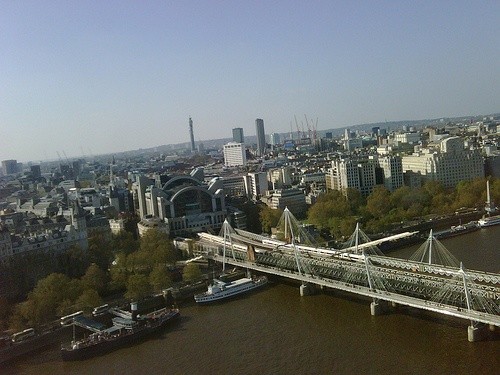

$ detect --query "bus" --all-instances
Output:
[60,310,83,325]
[92,303,109,315]
[10,327,34,342]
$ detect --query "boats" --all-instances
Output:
[432,221,481,239]
[62,292,178,361]
[194,272,268,304]
[478,215,500,227]
